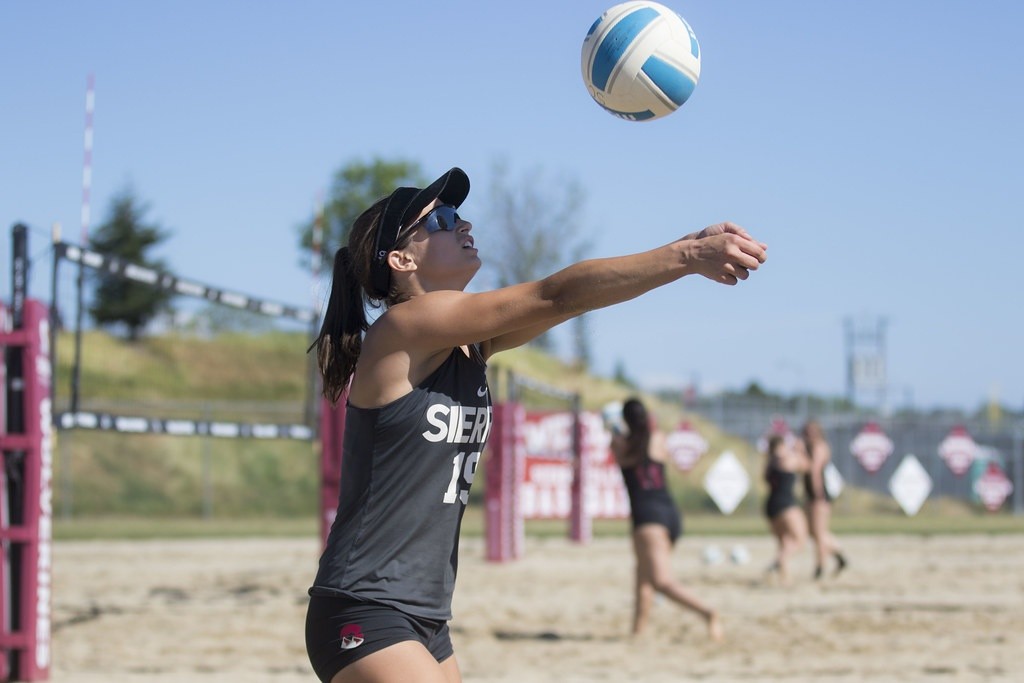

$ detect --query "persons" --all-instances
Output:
[306,176,768,683]
[608,394,719,641]
[765,418,848,584]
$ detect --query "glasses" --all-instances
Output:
[381,204,460,267]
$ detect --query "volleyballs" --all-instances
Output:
[580,1,703,123]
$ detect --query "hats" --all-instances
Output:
[368,165,471,301]
